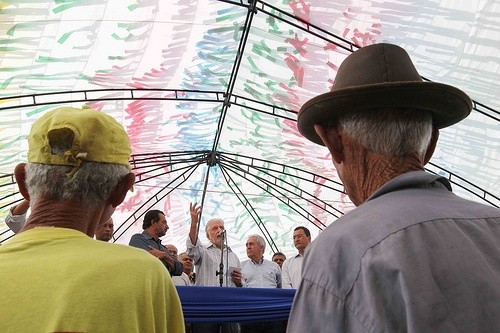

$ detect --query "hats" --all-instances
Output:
[297,44,472,145]
[27,105,131,165]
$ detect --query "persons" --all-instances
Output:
[129,210,184,277]
[161,244,195,286]
[282,226,312,289]
[4,198,30,235]
[272,252,286,268]
[93,217,114,242]
[0,106,185,333]
[240,234,282,288]
[186,202,242,287]
[284,42,500,333]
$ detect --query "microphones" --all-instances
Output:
[219,230,226,235]
